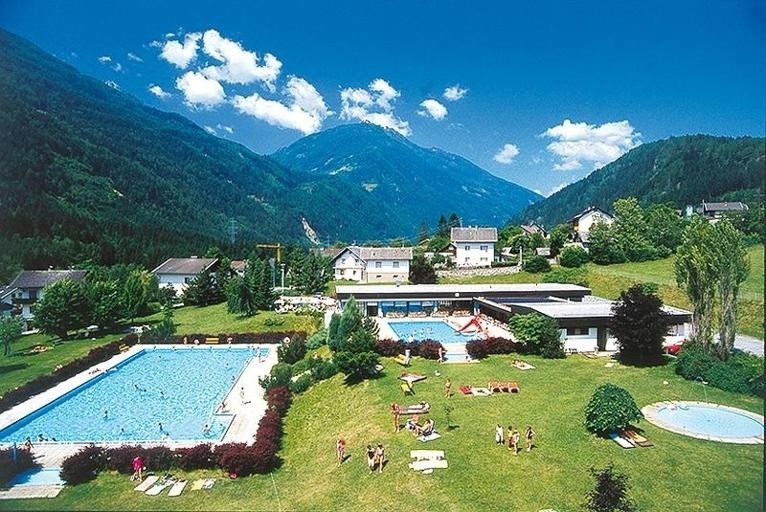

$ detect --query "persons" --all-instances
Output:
[391,399,434,439]
[437,345,444,363]
[23,436,32,453]
[495,421,536,457]
[38,433,55,442]
[409,326,432,342]
[239,386,244,404]
[201,423,211,435]
[336,439,344,466]
[375,442,384,473]
[464,349,468,359]
[365,445,376,471]
[445,379,452,398]
[131,453,144,482]
